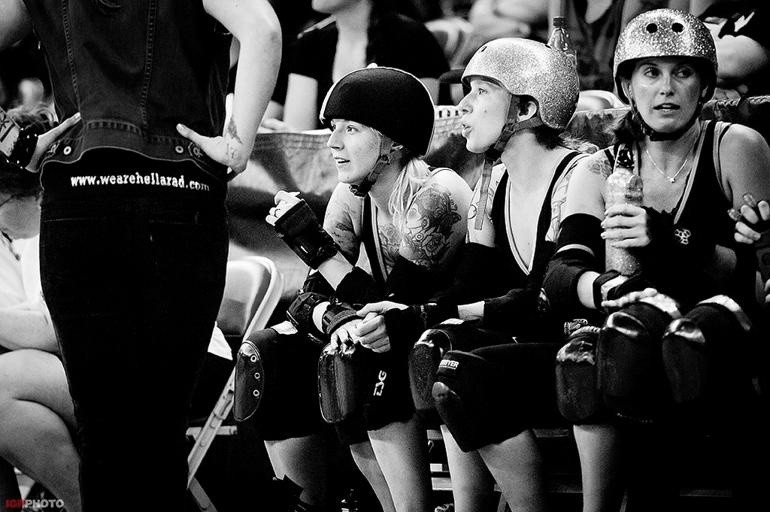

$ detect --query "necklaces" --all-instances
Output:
[638,143,699,185]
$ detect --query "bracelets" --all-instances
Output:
[5,126,39,172]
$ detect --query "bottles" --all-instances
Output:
[601,149,644,276]
[547,17,577,74]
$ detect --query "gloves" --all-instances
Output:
[275,199,340,269]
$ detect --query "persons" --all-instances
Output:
[3,259,254,511]
[231,59,475,512]
[351,37,594,512]
[424,6,769,512]
[0,0,290,511]
[594,186,768,511]
[0,153,63,354]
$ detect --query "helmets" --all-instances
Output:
[460,35,580,130]
[612,7,719,103]
[319,66,437,158]
